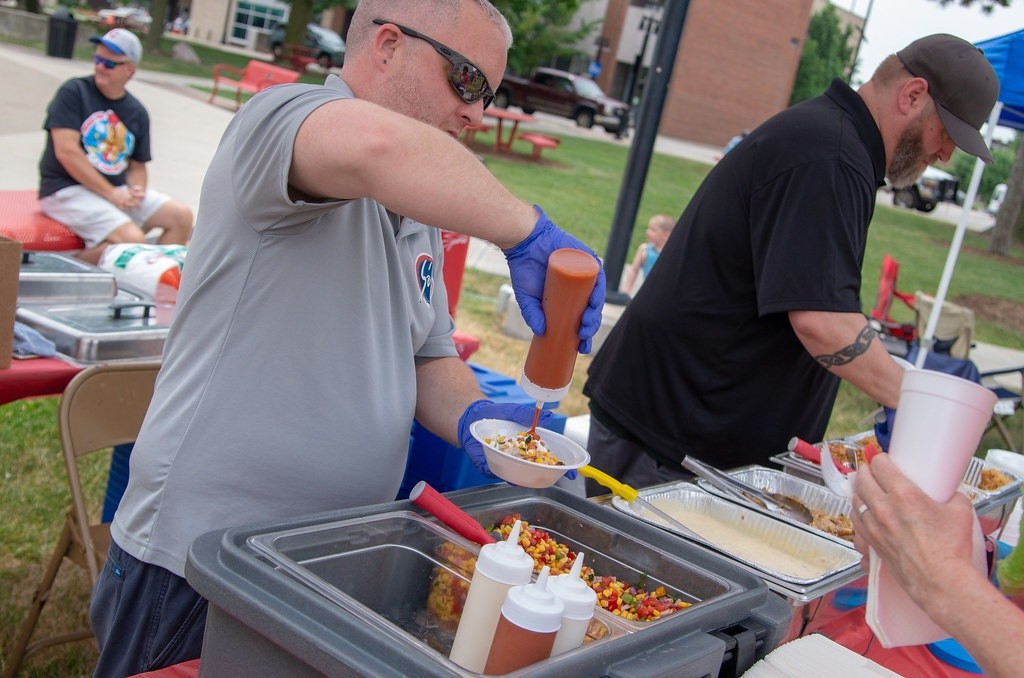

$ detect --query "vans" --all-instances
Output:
[988,184,1008,215]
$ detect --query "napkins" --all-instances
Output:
[738,633,906,678]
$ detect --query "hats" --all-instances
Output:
[87,29,144,68]
[896,31,1001,167]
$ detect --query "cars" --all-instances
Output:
[956,189,966,206]
[97,6,152,34]
[268,19,346,69]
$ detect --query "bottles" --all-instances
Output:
[449,519,535,675]
[518,248,601,408]
[485,565,566,675]
[548,552,597,660]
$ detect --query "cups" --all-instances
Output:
[888,367,1000,504]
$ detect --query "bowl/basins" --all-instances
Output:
[470,418,591,489]
[982,448,1024,548]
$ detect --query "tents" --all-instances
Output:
[913,22,1022,373]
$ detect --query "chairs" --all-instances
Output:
[7,359,162,678]
[861,253,1024,456]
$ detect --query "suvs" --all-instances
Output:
[883,164,959,214]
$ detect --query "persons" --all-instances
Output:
[85,0,608,678]
[38,29,192,265]
[586,31,996,499]
[621,213,676,297]
[852,453,1024,677]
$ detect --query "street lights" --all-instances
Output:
[615,14,662,142]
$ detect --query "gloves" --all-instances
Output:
[458,394,581,485]
[502,203,607,357]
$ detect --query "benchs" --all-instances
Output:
[0,189,84,250]
[207,58,302,111]
[463,121,560,161]
[283,43,340,75]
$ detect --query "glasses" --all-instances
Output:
[90,53,127,70]
[373,17,497,112]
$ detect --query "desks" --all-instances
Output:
[129,543,1023,677]
[969,342,1024,399]
[2,334,482,406]
[482,107,537,153]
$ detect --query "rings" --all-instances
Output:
[855,503,869,520]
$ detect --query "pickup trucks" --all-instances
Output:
[493,67,635,132]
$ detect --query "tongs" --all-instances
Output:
[680,454,814,526]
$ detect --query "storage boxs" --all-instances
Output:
[0,236,21,371]
[394,359,567,503]
[183,480,794,678]
[102,442,134,524]
